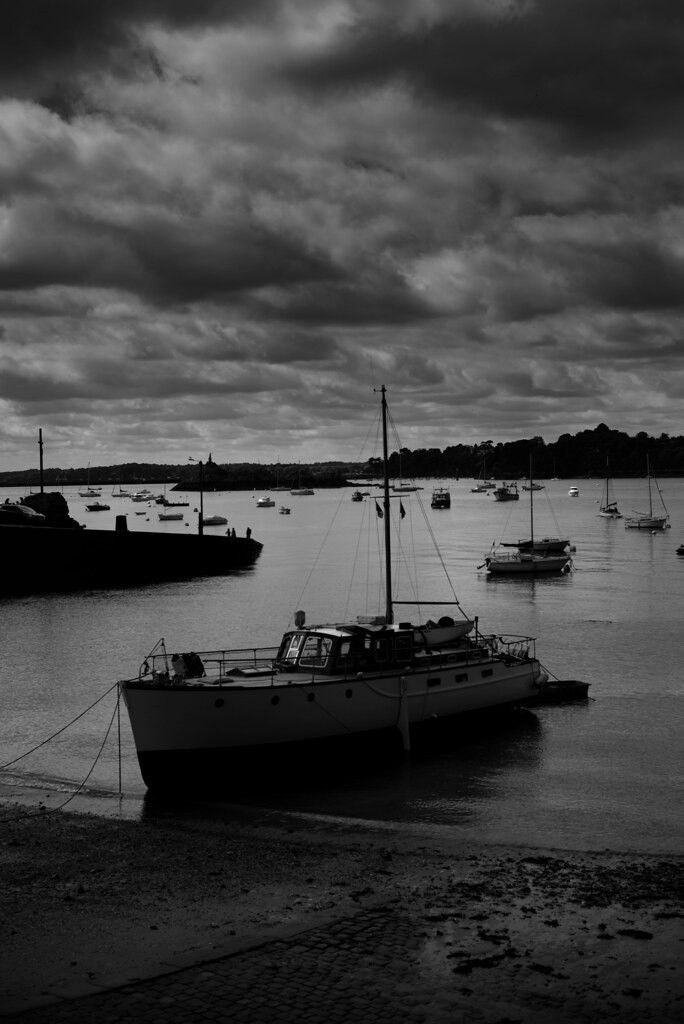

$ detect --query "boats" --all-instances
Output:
[352,491,363,501]
[278,506,290,514]
[256,497,275,507]
[492,481,519,501]
[536,681,592,704]
[522,483,545,490]
[568,487,578,497]
[430,487,450,508]
[414,620,475,647]
[77,461,228,526]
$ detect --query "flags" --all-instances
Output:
[375,500,383,519]
[400,500,406,519]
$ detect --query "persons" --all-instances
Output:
[226,527,251,538]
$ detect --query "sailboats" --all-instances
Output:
[379,455,424,492]
[290,469,314,495]
[471,456,496,492]
[624,454,670,529]
[551,463,559,480]
[270,469,292,491]
[477,452,570,574]
[117,384,541,759]
[599,456,623,518]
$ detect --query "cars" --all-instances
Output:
[0,504,45,526]
